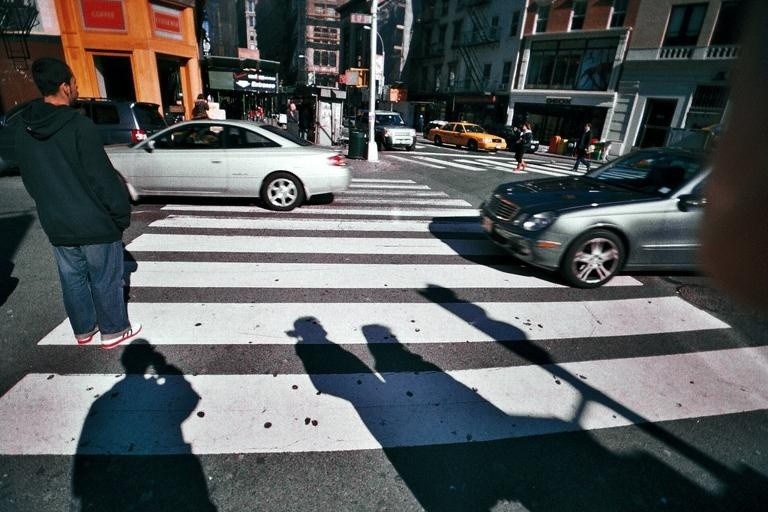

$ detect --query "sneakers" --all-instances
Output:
[78,321,142,350]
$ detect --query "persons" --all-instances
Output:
[247,105,263,121]
[191,93,209,119]
[419,115,424,132]
[287,103,300,136]
[569,122,592,171]
[513,122,533,170]
[698,1,768,312]
[1,56,143,350]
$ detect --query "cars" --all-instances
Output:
[474,146,720,290]
[494,124,541,153]
[428,119,508,154]
[103,117,355,212]
[423,119,448,140]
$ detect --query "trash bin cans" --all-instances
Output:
[348,127,368,160]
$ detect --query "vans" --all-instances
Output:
[0,95,170,177]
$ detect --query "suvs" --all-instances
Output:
[357,108,418,152]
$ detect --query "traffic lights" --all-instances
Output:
[345,70,360,86]
[389,88,400,103]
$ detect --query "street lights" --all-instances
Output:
[297,54,316,85]
[362,25,385,55]
